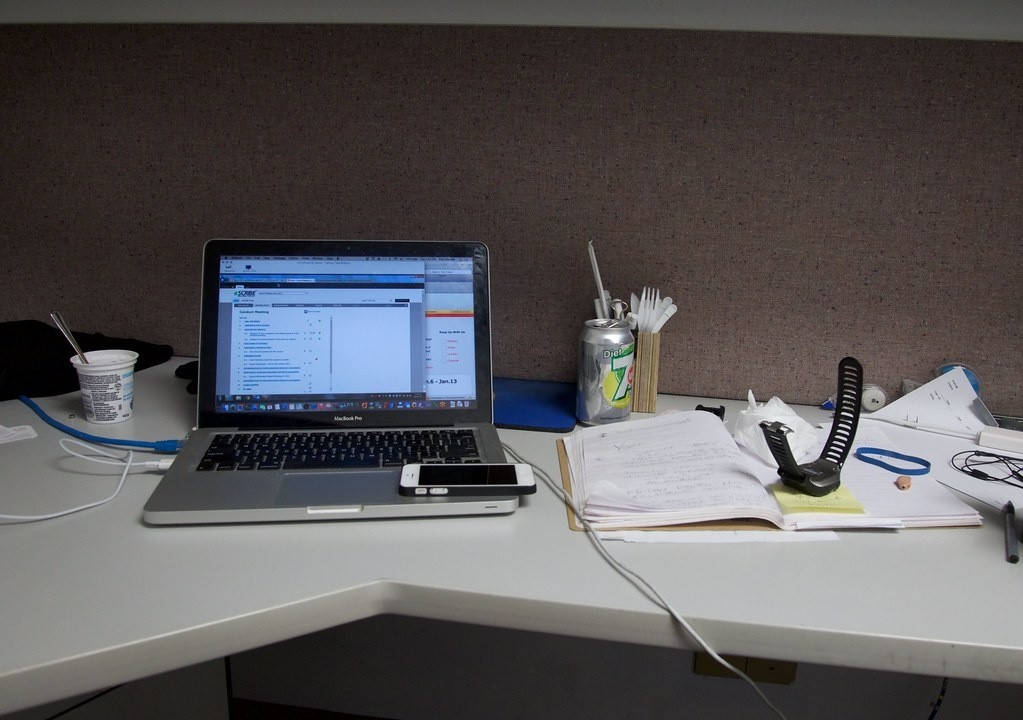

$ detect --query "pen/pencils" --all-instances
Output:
[1006,500,1019,563]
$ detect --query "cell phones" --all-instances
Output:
[397,464,538,497]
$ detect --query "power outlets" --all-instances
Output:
[694,652,798,686]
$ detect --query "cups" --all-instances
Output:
[70,349,139,424]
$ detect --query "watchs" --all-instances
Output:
[759,357,863,497]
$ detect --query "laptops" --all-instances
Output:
[142,239,518,525]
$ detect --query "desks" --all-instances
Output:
[0,348,1023,720]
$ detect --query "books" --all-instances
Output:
[557,409,982,542]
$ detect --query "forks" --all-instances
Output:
[637,287,660,333]
